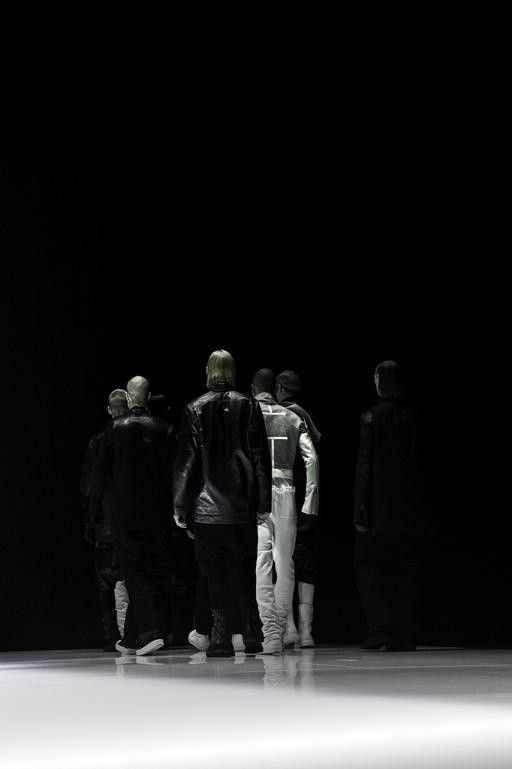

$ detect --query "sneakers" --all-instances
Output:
[360,636,415,651]
[188,629,209,651]
[115,628,165,655]
[231,633,246,652]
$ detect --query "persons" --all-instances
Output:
[352,358,442,654]
[77,347,326,655]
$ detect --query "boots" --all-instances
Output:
[260,603,314,652]
